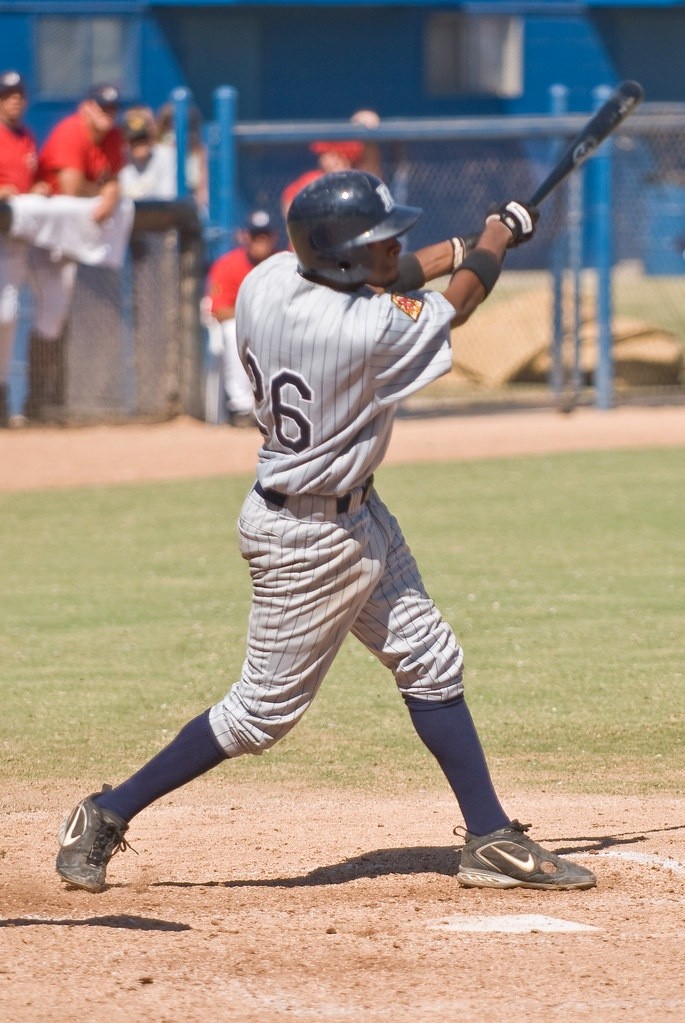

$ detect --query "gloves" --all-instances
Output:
[449,231,481,274]
[484,198,539,248]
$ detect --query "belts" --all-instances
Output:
[254,474,374,514]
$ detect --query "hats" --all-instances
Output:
[122,108,159,141]
[0,72,24,96]
[87,84,122,110]
[310,139,365,163]
[242,206,276,236]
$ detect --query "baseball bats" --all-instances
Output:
[526,78,645,208]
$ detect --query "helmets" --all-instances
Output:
[287,170,424,283]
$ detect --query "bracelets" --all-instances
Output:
[451,250,501,302]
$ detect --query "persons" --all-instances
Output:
[281,110,381,248]
[198,210,292,427]
[0,70,208,427]
[56,169,599,892]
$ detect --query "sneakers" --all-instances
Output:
[55,783,140,892]
[452,819,598,891]
